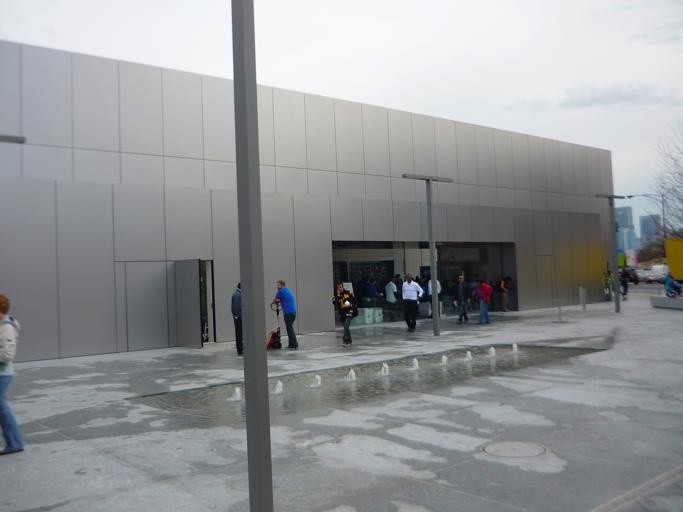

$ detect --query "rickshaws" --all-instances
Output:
[267,301,282,350]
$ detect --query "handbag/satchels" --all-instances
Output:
[351,295,359,318]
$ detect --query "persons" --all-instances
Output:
[500,275,514,312]
[0,294,25,455]
[271,279,299,349]
[230,281,244,356]
[401,273,424,333]
[330,279,355,346]
[361,270,500,326]
[663,270,683,298]
[620,268,630,296]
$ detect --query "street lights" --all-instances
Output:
[627,194,666,239]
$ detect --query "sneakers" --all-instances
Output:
[456,319,469,323]
[0,447,24,456]
[342,343,351,347]
[285,346,299,350]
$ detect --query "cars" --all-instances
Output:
[618,265,668,283]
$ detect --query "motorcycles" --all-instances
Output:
[666,276,683,297]
